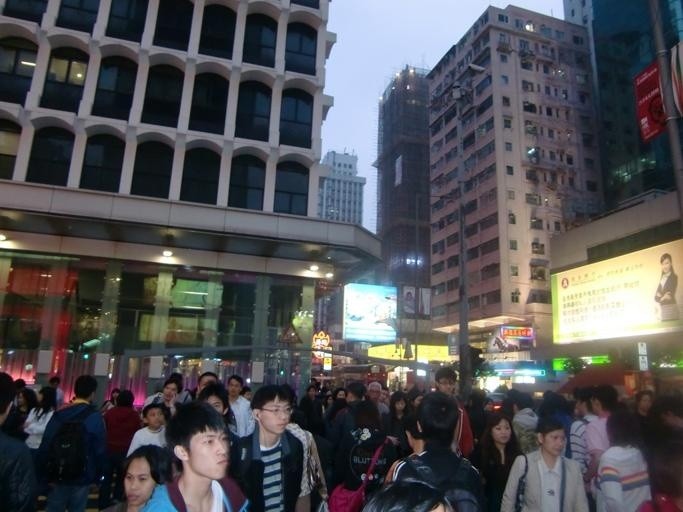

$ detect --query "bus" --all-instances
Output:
[335,363,386,389]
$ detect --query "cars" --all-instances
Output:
[487,392,508,412]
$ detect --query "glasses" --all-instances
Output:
[437,381,456,386]
[261,407,295,414]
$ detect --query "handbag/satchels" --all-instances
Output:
[500,478,525,512]
[328,482,365,511]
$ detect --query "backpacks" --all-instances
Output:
[43,421,88,484]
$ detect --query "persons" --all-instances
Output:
[404,291,414,312]
[0,368,681,511]
[654,252,680,321]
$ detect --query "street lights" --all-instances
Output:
[426,63,486,419]
[384,296,417,390]
